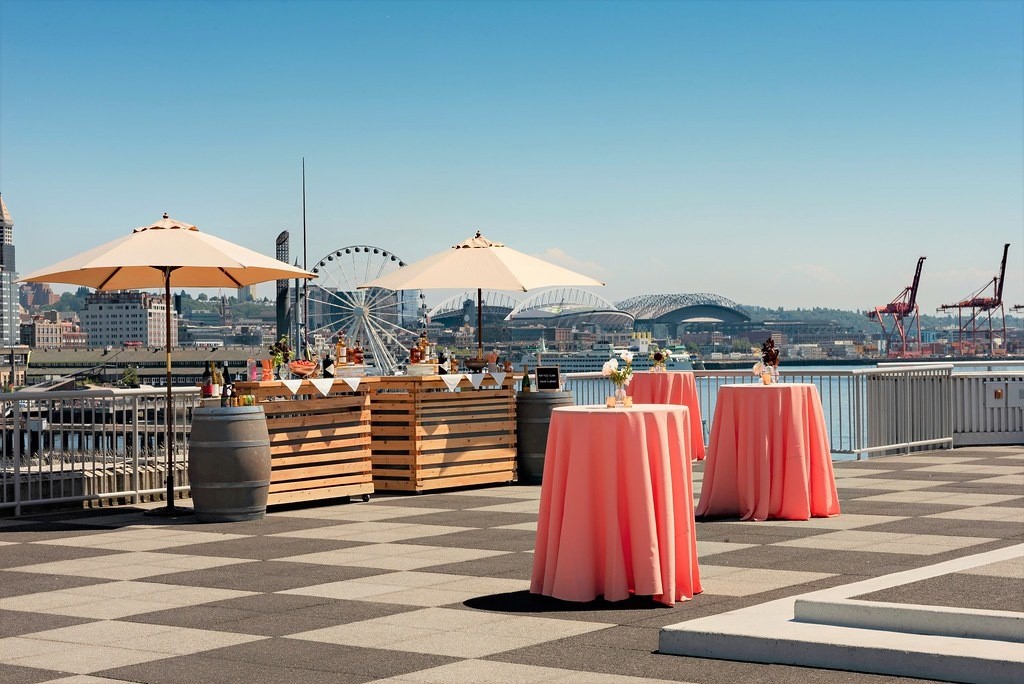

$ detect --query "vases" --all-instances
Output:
[771,366,780,383]
[656,365,661,372]
[615,390,627,405]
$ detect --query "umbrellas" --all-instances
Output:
[357,230,605,357]
[7,213,321,500]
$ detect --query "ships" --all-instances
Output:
[519,341,693,371]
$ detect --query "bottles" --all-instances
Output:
[234,372,242,392]
[218,361,225,395]
[202,361,212,397]
[353,339,364,366]
[522,365,530,393]
[344,338,355,367]
[323,355,334,378]
[221,383,230,406]
[247,346,257,382]
[336,331,347,366]
[410,331,458,375]
[210,361,219,398]
[222,361,233,397]
[231,383,238,407]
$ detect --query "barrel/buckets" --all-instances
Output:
[188,405,271,522]
[516,390,575,485]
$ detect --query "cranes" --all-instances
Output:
[936,241,1010,355]
[864,256,927,359]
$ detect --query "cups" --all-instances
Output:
[762,374,771,385]
[262,368,274,381]
[622,396,632,408]
[487,363,496,373]
[606,396,616,409]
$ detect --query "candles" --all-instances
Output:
[606,397,616,408]
[649,367,654,372]
[762,374,771,385]
[623,395,633,408]
[661,367,666,373]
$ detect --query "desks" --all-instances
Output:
[720,383,816,521]
[370,371,526,495]
[529,404,691,609]
[627,370,695,464]
[196,376,382,508]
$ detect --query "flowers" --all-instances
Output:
[751,337,780,376]
[647,343,673,365]
[600,350,634,388]
